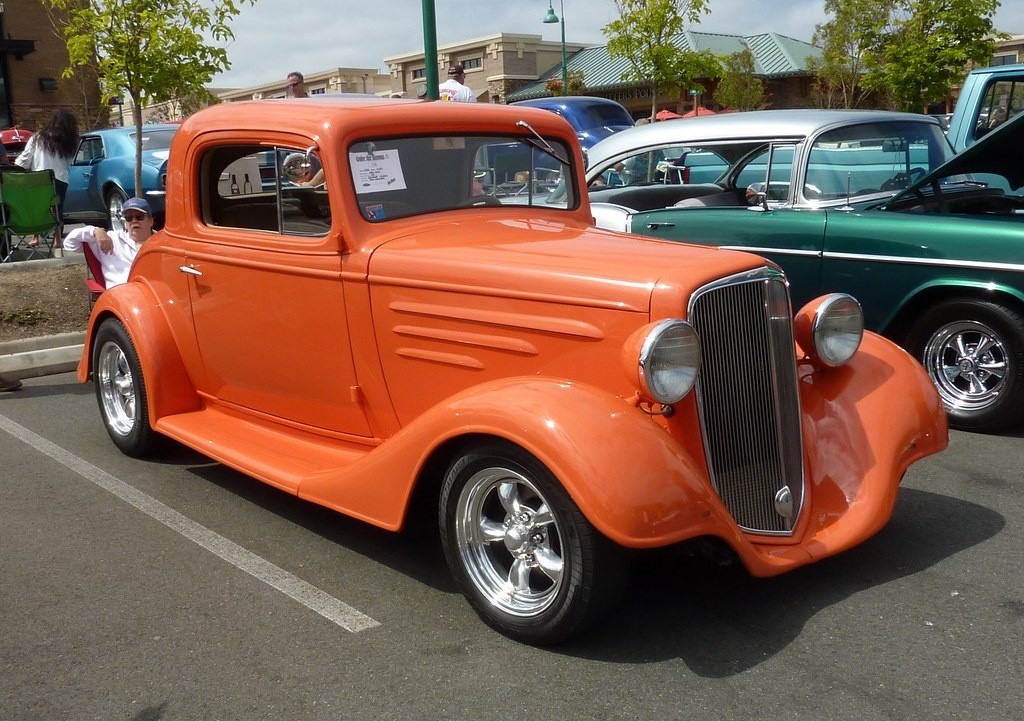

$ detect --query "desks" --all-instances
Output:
[499,181,556,196]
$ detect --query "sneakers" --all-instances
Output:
[42,231,55,238]
[61,233,69,238]
[28,238,39,246]
[53,239,64,248]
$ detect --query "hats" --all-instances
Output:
[635,118,649,127]
[416,83,427,96]
[474,170,487,177]
[121,197,152,216]
[448,65,464,74]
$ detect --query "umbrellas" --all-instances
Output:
[647,105,745,122]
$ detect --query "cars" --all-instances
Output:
[472,96,641,194]
[62,122,319,234]
[478,108,1023,435]
[76,99,949,651]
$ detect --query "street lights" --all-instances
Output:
[543,0,567,96]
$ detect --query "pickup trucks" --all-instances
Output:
[684,64,1024,198]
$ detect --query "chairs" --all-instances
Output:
[361,200,416,221]
[217,202,302,229]
[0,166,63,262]
[82,241,107,313]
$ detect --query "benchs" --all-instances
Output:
[677,186,753,208]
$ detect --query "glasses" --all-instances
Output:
[124,213,149,222]
[473,176,485,182]
[287,81,302,88]
[462,74,466,78]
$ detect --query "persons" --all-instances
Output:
[13,110,82,248]
[438,65,477,103]
[614,119,662,186]
[280,72,325,190]
[63,198,157,290]
[0,137,9,164]
